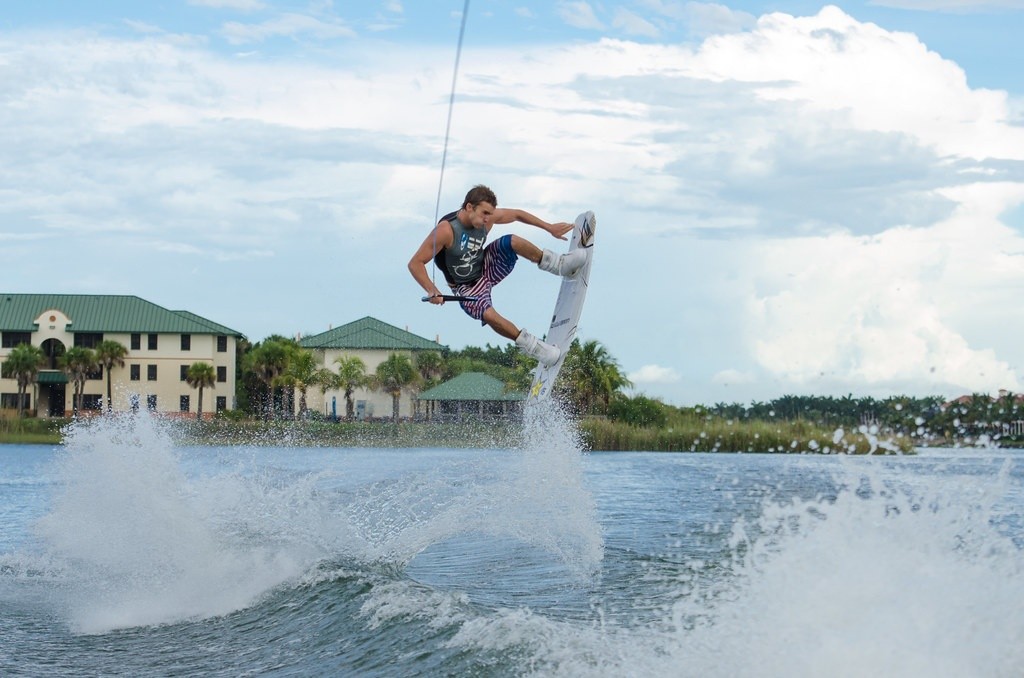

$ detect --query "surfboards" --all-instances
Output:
[525,210,597,407]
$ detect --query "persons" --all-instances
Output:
[407,185,587,365]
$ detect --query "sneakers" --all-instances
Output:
[538,248,588,278]
[515,328,561,366]
